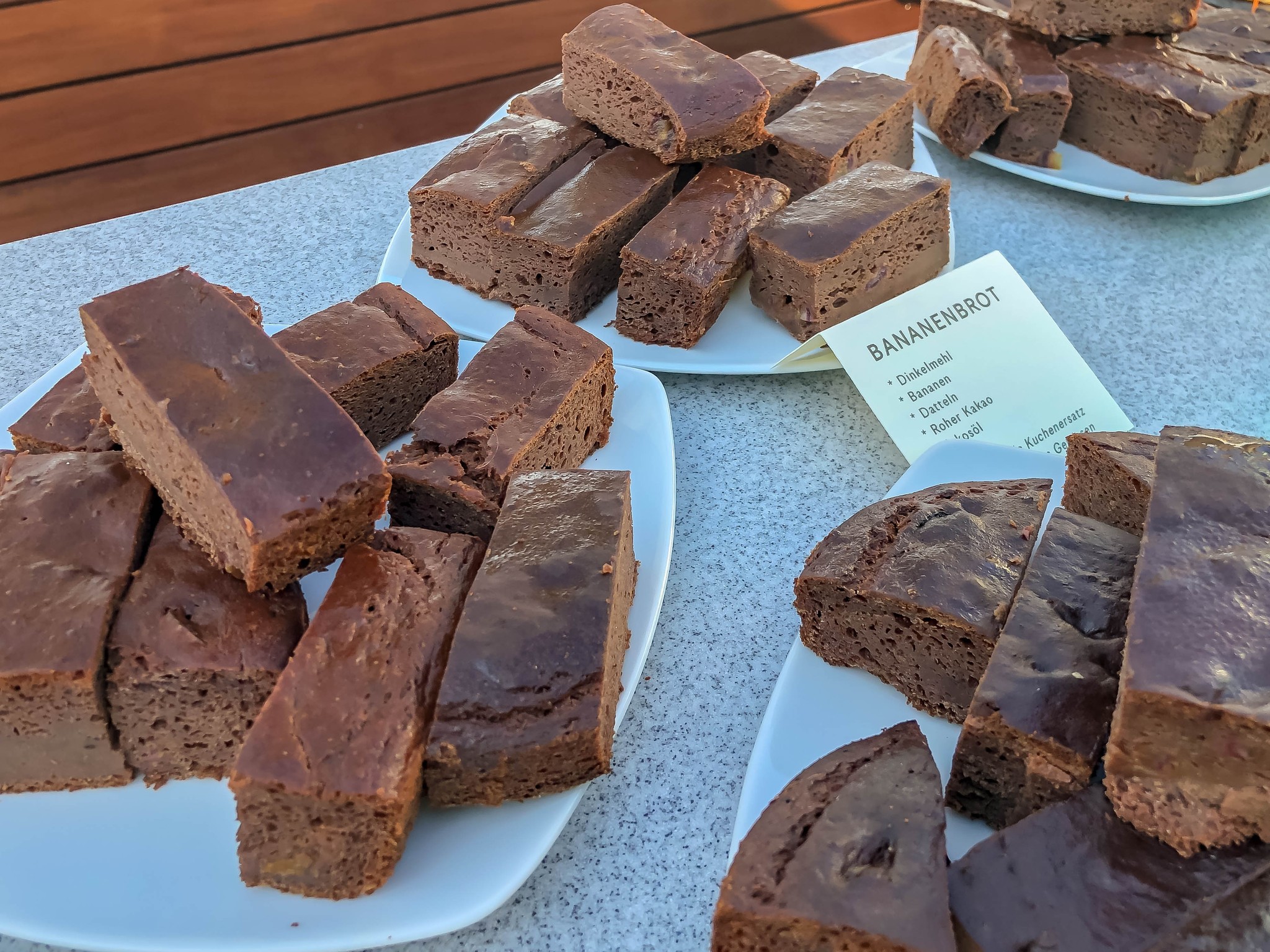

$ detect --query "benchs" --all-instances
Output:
[0,1,923,239]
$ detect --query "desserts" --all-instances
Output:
[405,2,950,346]
[708,423,1269,952]
[903,0,1270,185]
[0,263,639,899]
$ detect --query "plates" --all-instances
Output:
[724,440,1067,866]
[853,42,1270,207]
[0,324,677,952]
[375,130,956,374]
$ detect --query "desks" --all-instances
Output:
[1,28,1270,952]
